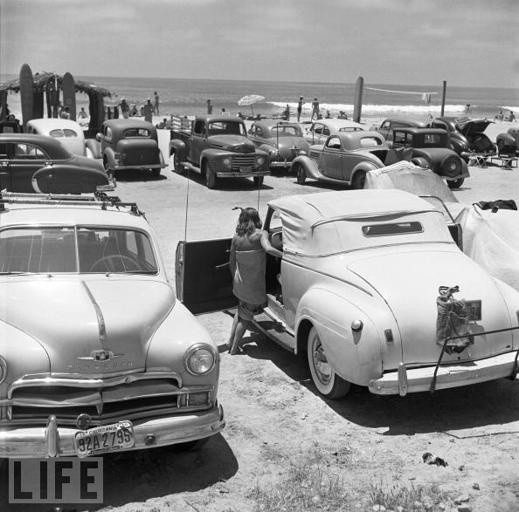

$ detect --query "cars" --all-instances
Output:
[0,188,225,459]
[0,116,496,193]
[174,187,519,400]
[497,127,518,156]
[364,159,518,293]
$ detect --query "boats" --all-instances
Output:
[236,93,267,108]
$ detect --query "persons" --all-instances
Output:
[206,100,212,114]
[59,105,87,121]
[503,112,516,122]
[286,96,347,122]
[494,110,506,120]
[107,91,168,129]
[224,207,283,355]
